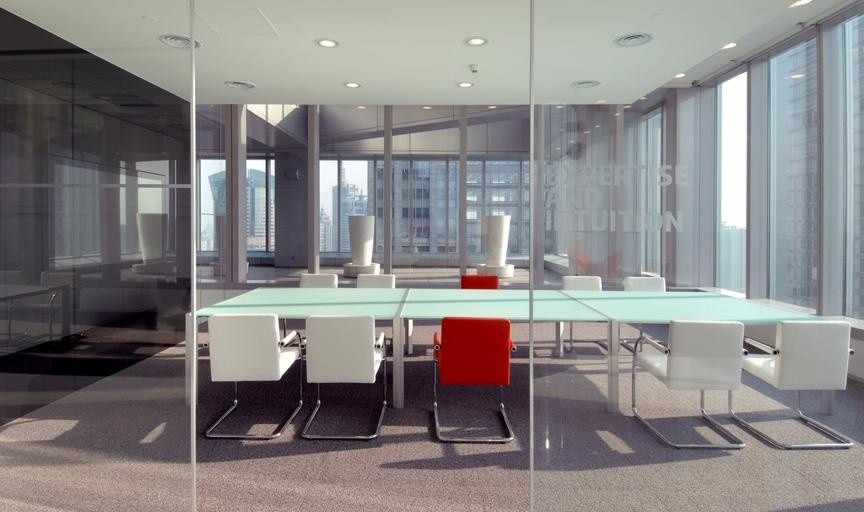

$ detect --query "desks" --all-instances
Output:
[181,286,406,420]
[0,281,73,356]
[392,288,617,415]
[553,287,842,416]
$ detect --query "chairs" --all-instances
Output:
[560,273,621,355]
[0,267,33,345]
[282,272,339,341]
[354,271,396,288]
[201,313,304,443]
[628,319,748,451]
[297,313,391,442]
[5,267,88,348]
[428,315,517,444]
[459,274,500,289]
[622,275,670,355]
[728,316,857,451]
